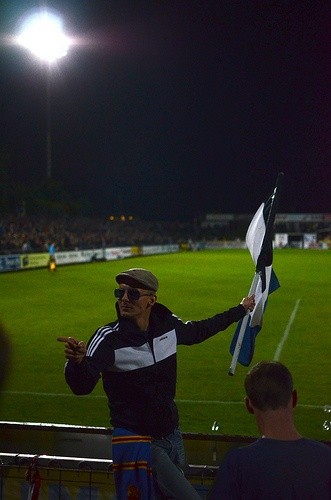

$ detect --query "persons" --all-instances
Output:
[0,212,223,256]
[208,360,331,499]
[54,268,256,500]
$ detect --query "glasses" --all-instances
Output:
[114,289,155,299]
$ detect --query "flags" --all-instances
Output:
[229,191,281,367]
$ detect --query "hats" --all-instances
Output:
[115,268,159,292]
